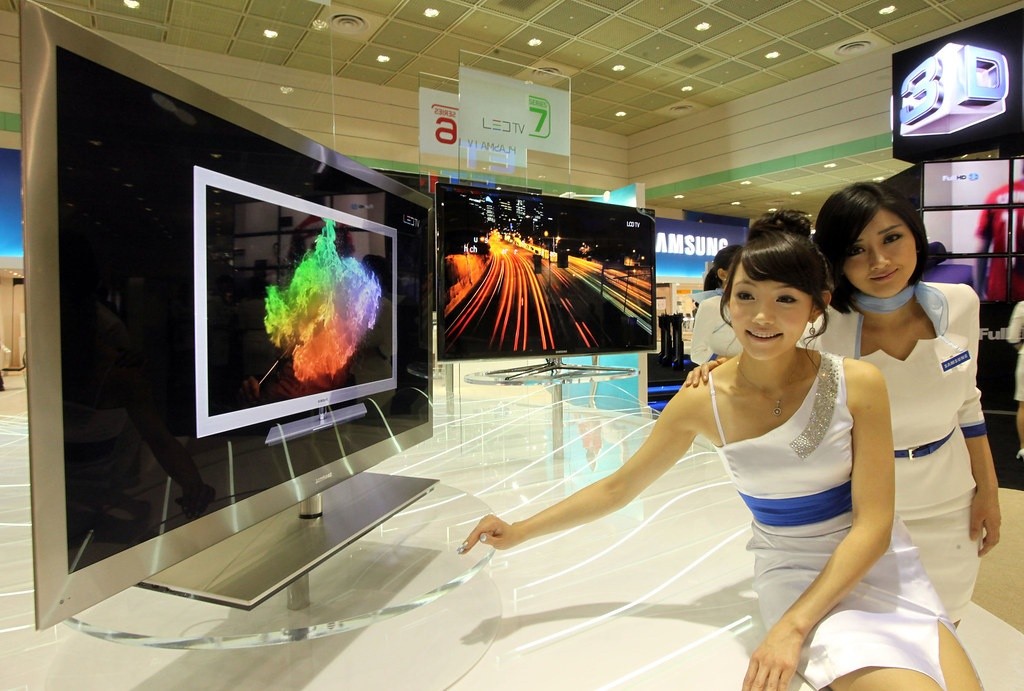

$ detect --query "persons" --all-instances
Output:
[976,161,1023,300]
[456,206,984,689]
[683,176,1004,636]
[688,244,744,366]
[1006,297,1024,460]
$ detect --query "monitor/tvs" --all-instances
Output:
[194,165,399,440]
[434,181,657,363]
[892,8,1024,164]
[14,0,434,633]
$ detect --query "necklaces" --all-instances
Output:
[736,348,799,417]
